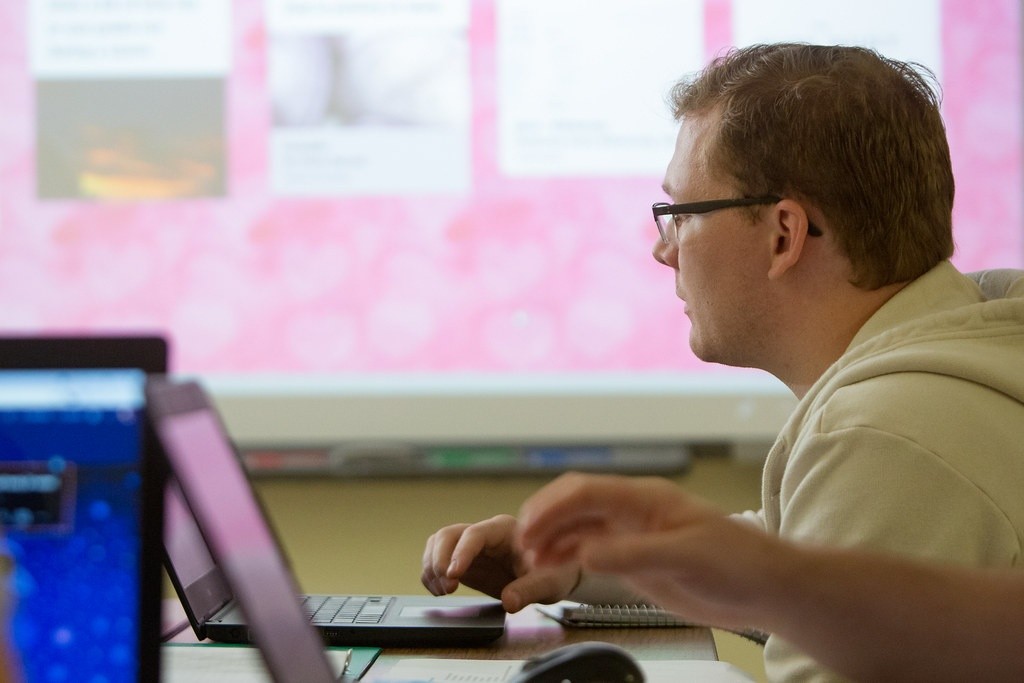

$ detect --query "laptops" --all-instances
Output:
[0,331,507,683]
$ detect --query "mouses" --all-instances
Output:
[517,641,644,683]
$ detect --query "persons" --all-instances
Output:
[514,470,1024,683]
[420,42,1024,683]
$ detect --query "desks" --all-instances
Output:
[162,599,717,661]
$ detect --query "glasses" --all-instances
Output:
[652,195,823,253]
